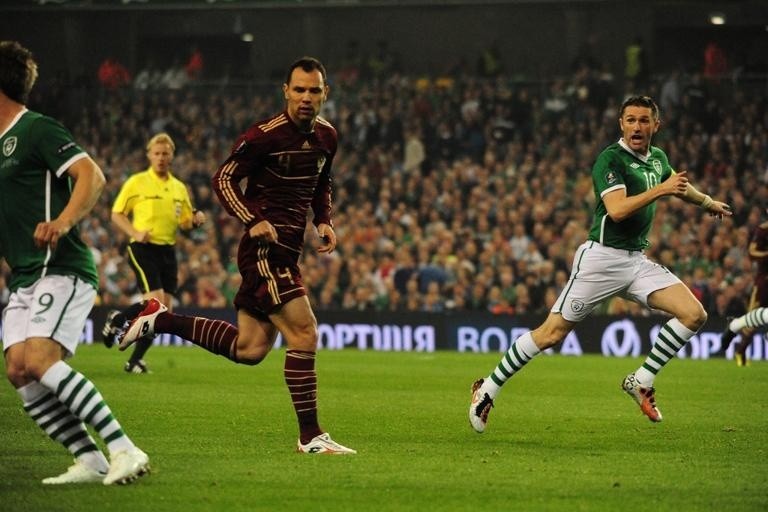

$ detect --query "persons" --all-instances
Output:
[0,23,768,363]
[0,40,152,488]
[468,95,734,435]
[104,135,194,373]
[115,57,357,455]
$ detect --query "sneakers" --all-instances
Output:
[124,360,149,373]
[622,371,662,422]
[735,340,745,367]
[103,444,150,487]
[117,297,168,350]
[468,377,492,434]
[721,316,736,351]
[41,457,109,485]
[297,432,357,455]
[102,309,121,348]
